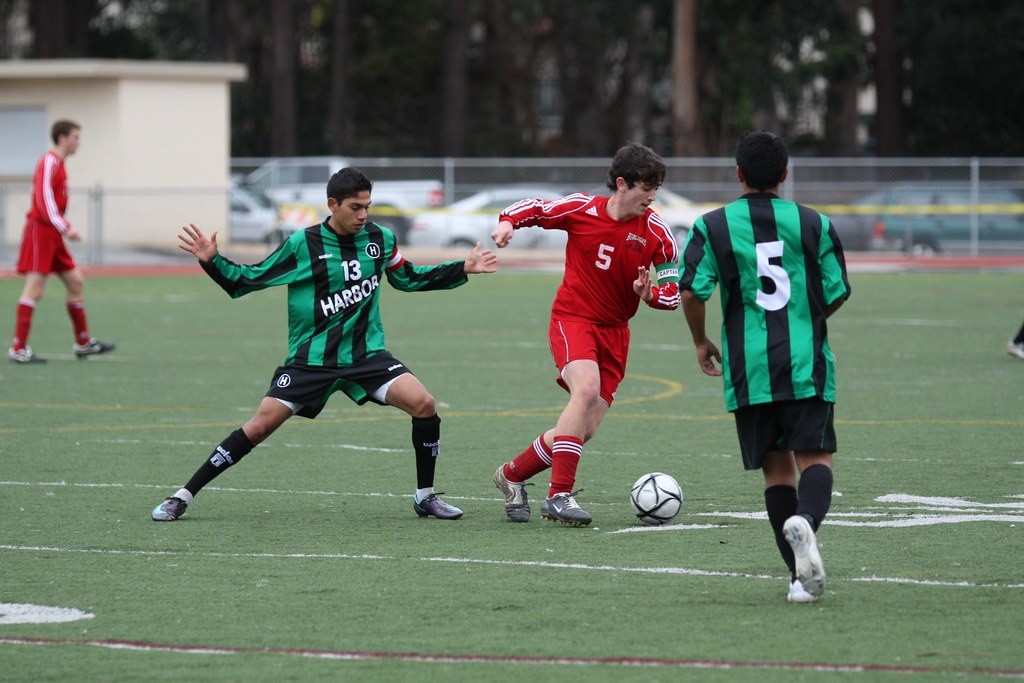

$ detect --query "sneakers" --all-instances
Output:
[493,463,535,523]
[72,338,116,360]
[8,344,47,364]
[413,492,463,519]
[152,496,188,521]
[540,489,592,527]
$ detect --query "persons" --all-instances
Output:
[490,143,681,526]
[8,119,115,363]
[153,168,498,523]
[679,132,853,602]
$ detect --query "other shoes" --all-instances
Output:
[787,579,819,602]
[782,515,825,597]
[1007,341,1024,360]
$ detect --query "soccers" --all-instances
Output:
[629,471,685,526]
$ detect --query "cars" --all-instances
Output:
[229,157,717,251]
[870,187,1024,257]
